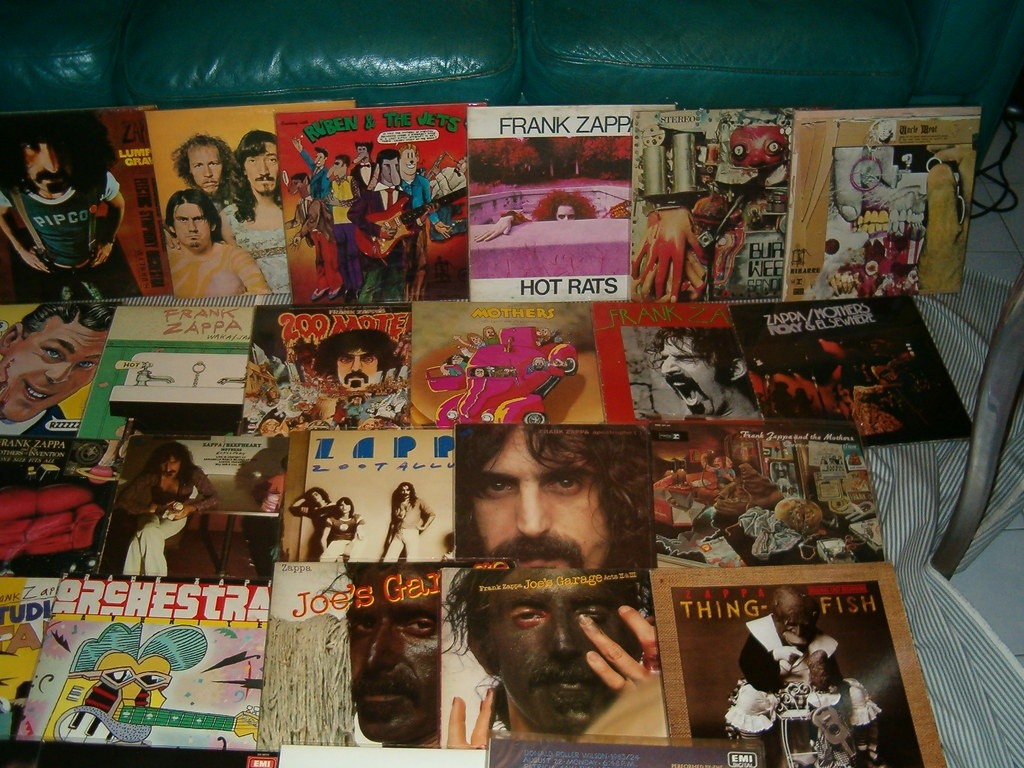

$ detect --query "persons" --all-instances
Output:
[473,190,632,243]
[162,129,290,297]
[727,590,885,768]
[453,425,645,570]
[450,568,660,750]
[340,563,442,748]
[114,443,216,578]
[647,326,758,427]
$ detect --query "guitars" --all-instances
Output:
[812,705,860,768]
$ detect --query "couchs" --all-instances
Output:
[0,0,1024,768]
